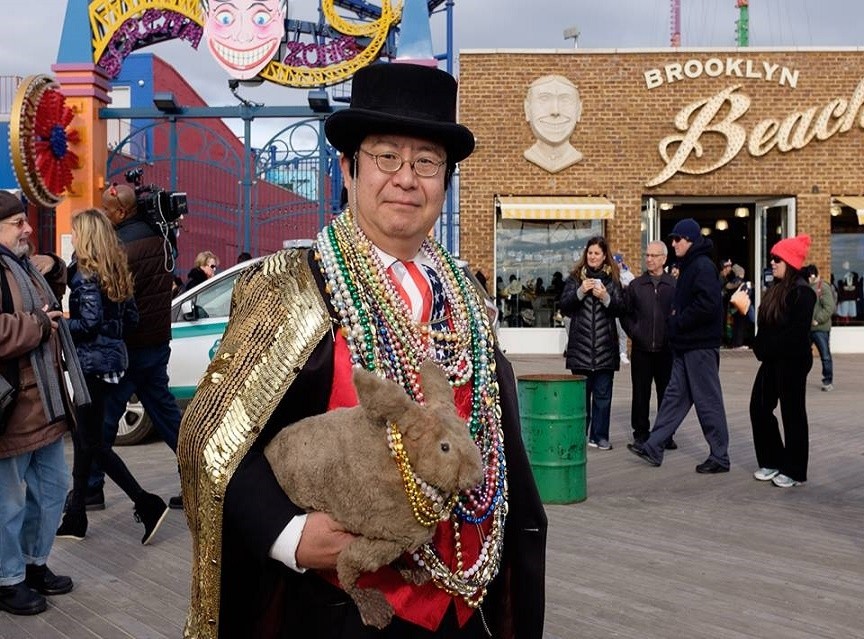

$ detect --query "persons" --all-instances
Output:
[54,206,170,546]
[173,251,252,299]
[832,262,858,317]
[560,236,623,449]
[0,190,75,617]
[66,183,182,510]
[182,64,547,639]
[620,221,839,487]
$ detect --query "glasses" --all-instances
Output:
[358,147,447,177]
[672,236,690,242]
[0,217,30,228]
[771,255,781,263]
[110,183,127,210]
[205,264,215,269]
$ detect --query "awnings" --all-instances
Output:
[831,196,864,225]
[496,196,615,220]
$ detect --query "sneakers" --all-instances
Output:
[772,474,803,487]
[754,467,780,480]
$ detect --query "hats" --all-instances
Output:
[508,280,523,294]
[770,234,812,271]
[668,218,700,241]
[324,64,475,166]
[0,190,25,221]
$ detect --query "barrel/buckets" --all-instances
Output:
[517,374,587,504]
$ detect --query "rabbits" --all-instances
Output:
[263,355,482,630]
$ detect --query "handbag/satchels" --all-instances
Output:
[729,282,751,315]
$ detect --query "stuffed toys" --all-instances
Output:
[266,358,484,629]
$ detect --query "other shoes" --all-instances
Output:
[62,483,106,511]
[54,505,88,540]
[0,581,48,617]
[23,561,73,596]
[131,490,169,545]
[822,384,833,392]
[168,495,185,510]
[588,429,730,475]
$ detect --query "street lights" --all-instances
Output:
[562,26,582,50]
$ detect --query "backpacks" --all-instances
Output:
[816,280,838,308]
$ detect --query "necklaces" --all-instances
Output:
[315,205,508,605]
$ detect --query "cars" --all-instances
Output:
[112,237,314,447]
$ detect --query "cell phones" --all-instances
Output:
[47,303,63,322]
[592,279,601,289]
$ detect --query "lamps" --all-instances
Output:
[153,93,180,114]
[228,79,251,107]
[701,227,711,236]
[307,90,330,110]
[734,206,749,218]
[716,219,728,231]
